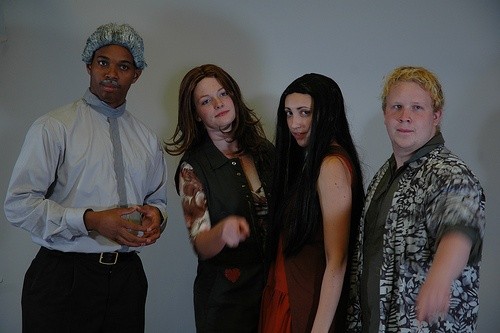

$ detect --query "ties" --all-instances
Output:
[83,87,128,207]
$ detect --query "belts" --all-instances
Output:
[55,251,139,265]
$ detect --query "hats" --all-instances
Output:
[82,23,145,74]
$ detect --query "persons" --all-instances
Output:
[5,23,168,333]
[345,67,486,333]
[263,72,365,333]
[164,63,279,333]
